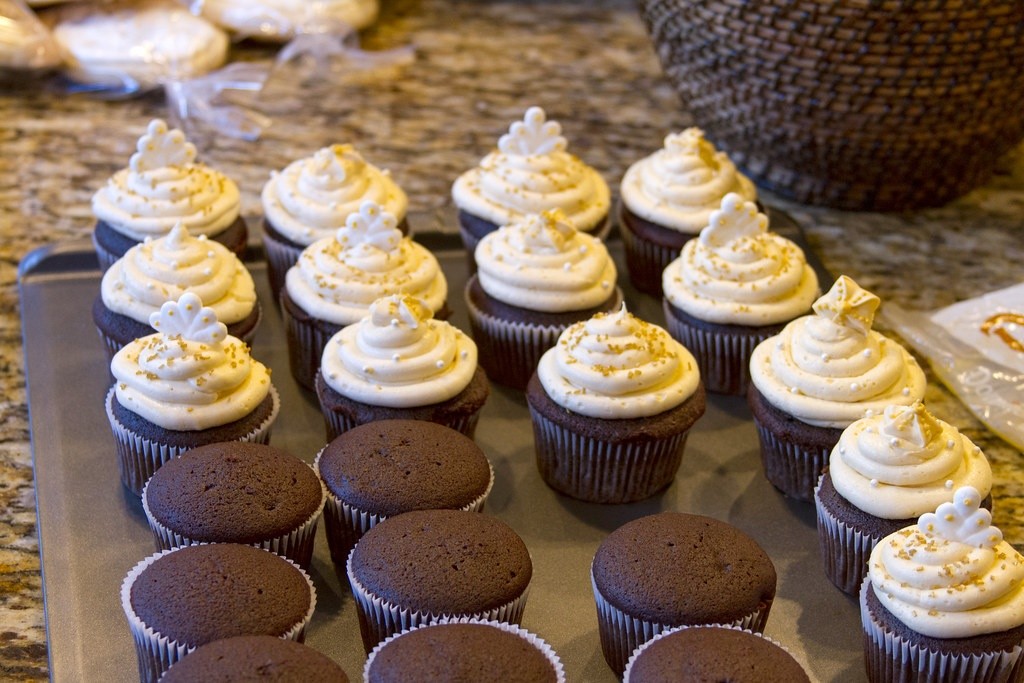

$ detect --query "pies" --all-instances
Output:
[0,0,377,86]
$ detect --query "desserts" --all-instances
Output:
[92,107,1024,683]
